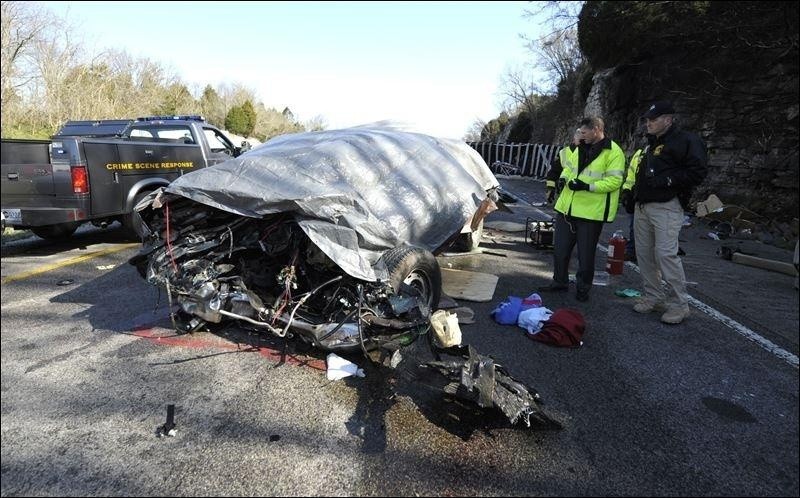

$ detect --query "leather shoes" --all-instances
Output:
[577,289,590,301]
[538,284,569,292]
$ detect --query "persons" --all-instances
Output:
[541,126,582,208]
[621,144,686,261]
[631,101,711,326]
[533,115,627,303]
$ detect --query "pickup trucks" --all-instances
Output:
[0,114,248,244]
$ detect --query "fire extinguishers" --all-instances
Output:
[606,230,625,275]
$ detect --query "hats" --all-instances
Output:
[640,102,675,121]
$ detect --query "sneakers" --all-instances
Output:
[633,301,668,313]
[660,306,690,323]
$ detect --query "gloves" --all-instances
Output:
[568,178,589,190]
[621,190,634,209]
